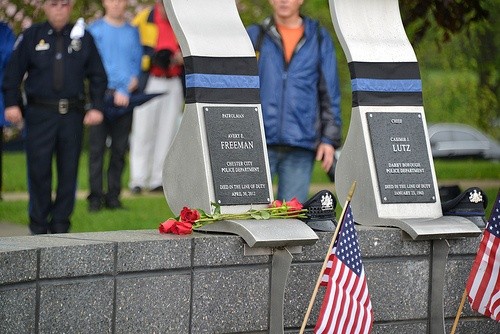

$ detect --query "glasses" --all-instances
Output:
[45,0,71,7]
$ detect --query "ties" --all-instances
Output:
[52,32,65,97]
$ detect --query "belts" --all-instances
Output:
[26,98,86,115]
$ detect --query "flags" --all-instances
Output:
[466,190,500,323]
[313,205,373,334]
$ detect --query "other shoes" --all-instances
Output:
[156,187,163,192]
[134,186,141,194]
[88,197,100,211]
[105,197,123,209]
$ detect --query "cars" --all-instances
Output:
[428,122,500,161]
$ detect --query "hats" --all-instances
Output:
[296,190,338,232]
[441,187,488,227]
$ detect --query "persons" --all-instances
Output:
[246,0,342,205]
[128,0,184,195]
[1,0,102,235]
[0,20,15,200]
[85,0,142,212]
